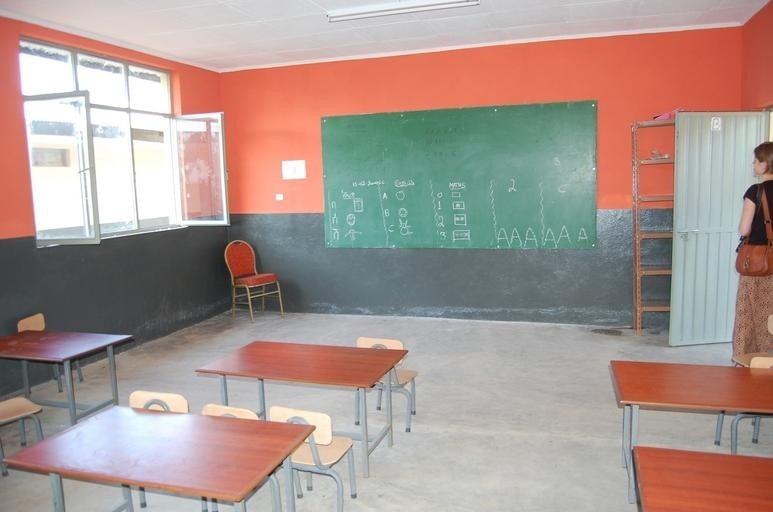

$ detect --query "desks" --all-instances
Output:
[195,340,409,478]
[0,404,317,511]
[610,359,773,504]
[0,330,134,425]
[631,445,773,512]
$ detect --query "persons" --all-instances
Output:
[731,141,773,370]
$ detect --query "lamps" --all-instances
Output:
[326,0,479,23]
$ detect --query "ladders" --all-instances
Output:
[630,119,675,336]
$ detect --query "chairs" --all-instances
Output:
[715,314,773,445]
[270,405,357,511]
[224,240,285,323]
[354,337,418,433]
[130,391,187,508]
[17,312,84,392]
[200,404,281,511]
[714,357,773,456]
[0,397,44,476]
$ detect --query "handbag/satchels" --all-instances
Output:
[735,243,773,276]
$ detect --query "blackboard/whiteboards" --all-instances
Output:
[319,100,597,249]
